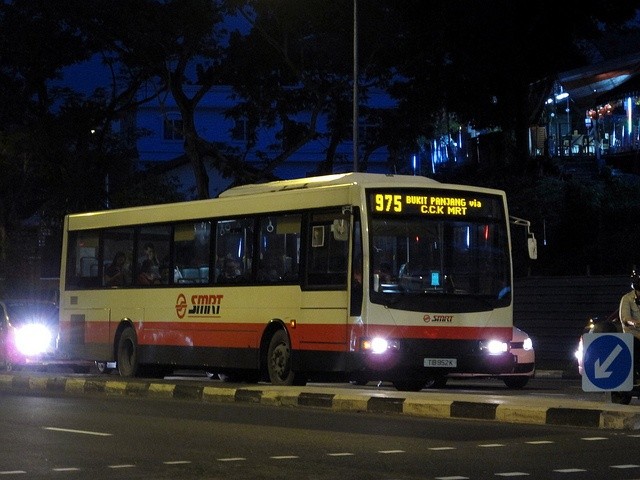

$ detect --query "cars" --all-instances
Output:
[349,323,536,389]
[0,299,90,374]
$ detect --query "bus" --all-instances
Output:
[58,172,538,392]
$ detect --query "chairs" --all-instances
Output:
[80,257,97,277]
[199,267,209,283]
[183,269,199,282]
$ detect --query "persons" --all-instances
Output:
[139,267,161,287]
[619,264,640,370]
[398,258,424,290]
[254,265,274,281]
[159,265,175,286]
[217,256,241,283]
[137,242,160,274]
[105,251,131,288]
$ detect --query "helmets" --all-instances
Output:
[631,269,640,291]
